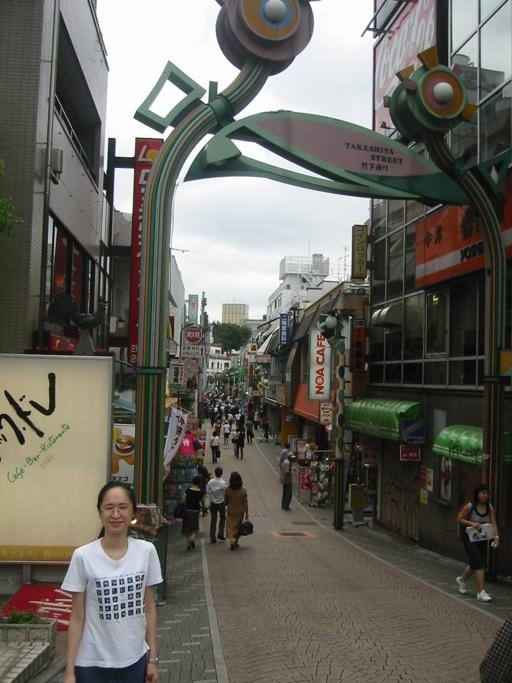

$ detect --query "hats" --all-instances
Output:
[287,452,296,459]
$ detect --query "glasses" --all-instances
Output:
[99,504,134,514]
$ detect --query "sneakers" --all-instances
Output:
[476,590,493,602]
[186,536,240,552]
[455,576,468,595]
[282,507,292,512]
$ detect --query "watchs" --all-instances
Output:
[147,656,159,664]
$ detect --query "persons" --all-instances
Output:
[453,483,501,602]
[179,386,298,551]
[59,482,165,683]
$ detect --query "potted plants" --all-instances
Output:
[0,607,55,666]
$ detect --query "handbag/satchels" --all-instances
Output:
[456,500,473,540]
[232,438,239,443]
[241,518,254,536]
[217,448,220,457]
[279,474,292,485]
